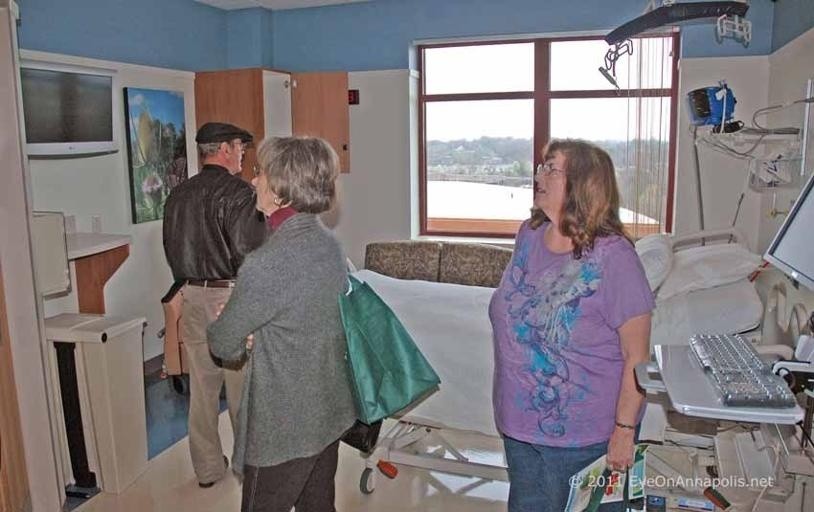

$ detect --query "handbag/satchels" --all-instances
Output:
[339,275,440,452]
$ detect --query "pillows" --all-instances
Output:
[632,232,765,301]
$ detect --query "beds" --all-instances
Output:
[339,226,783,493]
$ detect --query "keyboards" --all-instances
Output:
[688,332,795,408]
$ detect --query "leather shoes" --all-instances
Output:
[198,455,228,487]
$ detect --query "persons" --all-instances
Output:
[488,139,655,512]
[208,136,356,512]
[163,122,266,487]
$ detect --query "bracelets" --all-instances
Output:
[614,420,635,431]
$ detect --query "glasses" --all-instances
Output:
[255,167,267,179]
[537,164,566,176]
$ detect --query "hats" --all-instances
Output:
[195,122,253,144]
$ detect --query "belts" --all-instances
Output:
[188,278,236,288]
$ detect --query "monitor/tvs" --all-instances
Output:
[763,175,814,297]
[20,61,122,156]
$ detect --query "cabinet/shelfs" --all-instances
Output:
[195,66,351,182]
